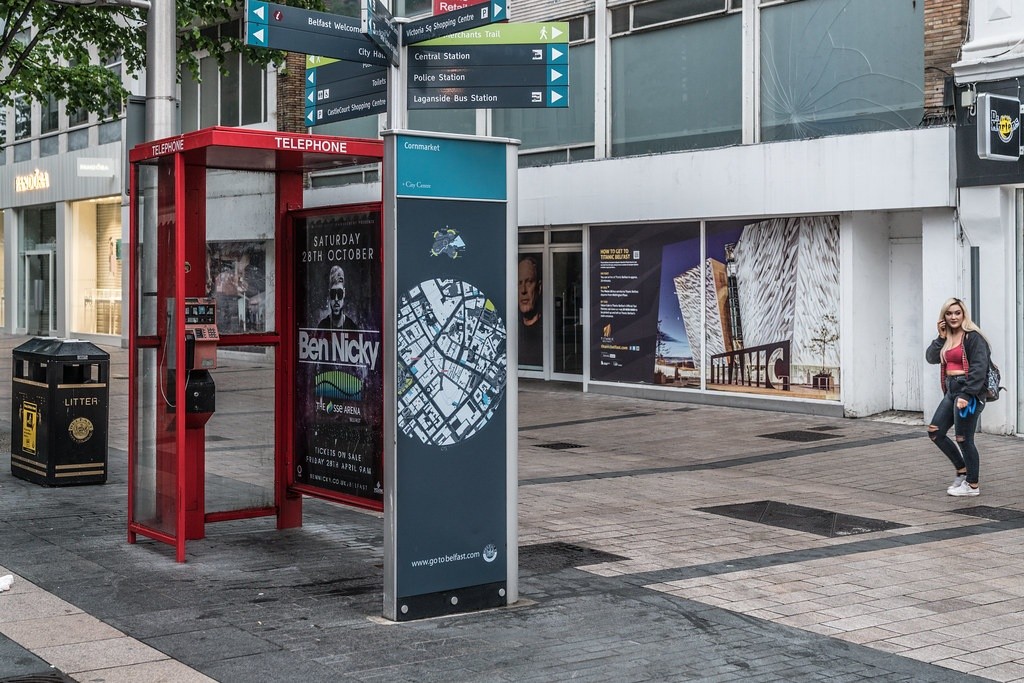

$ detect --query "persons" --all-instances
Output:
[318,266,359,330]
[213,250,266,332]
[926,299,991,497]
[518,257,543,366]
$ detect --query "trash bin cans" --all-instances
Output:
[9,336,110,487]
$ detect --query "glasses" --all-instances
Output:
[330,288,343,300]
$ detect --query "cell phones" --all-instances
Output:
[941,318,945,331]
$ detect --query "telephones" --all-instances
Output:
[166,297,219,370]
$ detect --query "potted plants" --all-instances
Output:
[654,320,667,384]
[803,314,840,390]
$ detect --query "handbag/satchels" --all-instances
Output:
[965,331,1007,402]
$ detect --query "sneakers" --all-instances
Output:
[947,474,966,490]
[947,480,980,497]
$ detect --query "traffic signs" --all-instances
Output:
[304,59,387,89]
[405,43,570,66]
[359,0,402,69]
[242,21,391,68]
[409,22,571,45]
[303,89,387,128]
[406,85,571,109]
[303,53,342,70]
[243,0,372,42]
[399,0,510,48]
[304,69,387,109]
[407,64,572,89]
